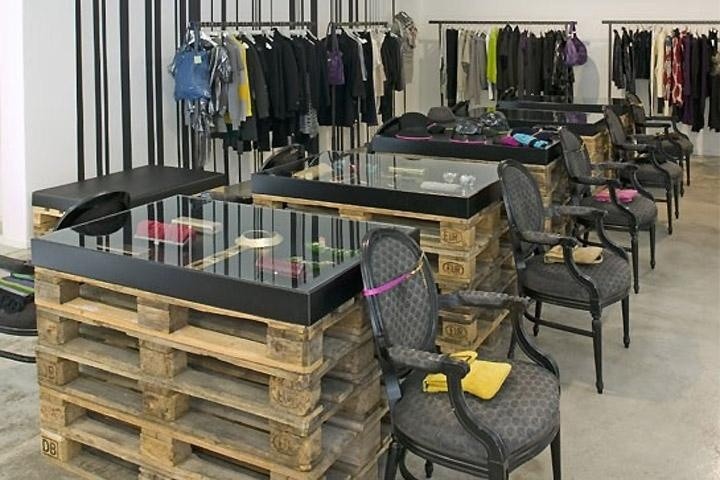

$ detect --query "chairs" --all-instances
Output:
[556,93,692,294]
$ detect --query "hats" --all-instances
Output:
[375,105,510,143]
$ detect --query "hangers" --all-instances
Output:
[188,19,399,50]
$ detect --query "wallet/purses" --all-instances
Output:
[94,241,152,259]
[170,216,225,235]
[291,162,333,180]
[256,256,305,279]
[133,219,197,246]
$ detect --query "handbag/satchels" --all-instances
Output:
[223,179,254,204]
[566,35,587,65]
[325,48,346,85]
[544,244,605,265]
[175,49,213,99]
[423,349,512,400]
[597,187,638,203]
[637,152,665,164]
